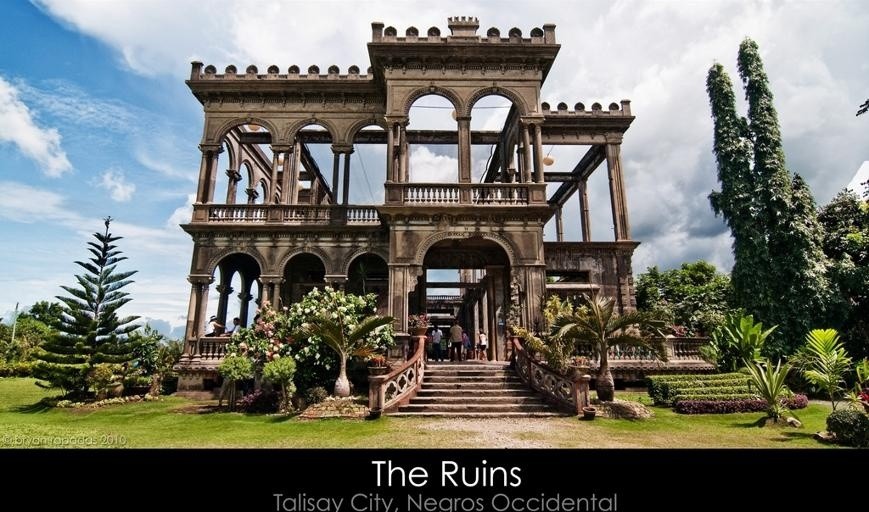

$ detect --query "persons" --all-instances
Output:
[205,315,226,337]
[431,319,487,362]
[228,318,243,337]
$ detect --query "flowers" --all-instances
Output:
[571,355,591,365]
[407,312,432,328]
[366,353,388,367]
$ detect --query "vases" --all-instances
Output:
[411,327,428,335]
[367,366,387,375]
[368,405,383,419]
[582,406,597,420]
[572,366,590,375]
